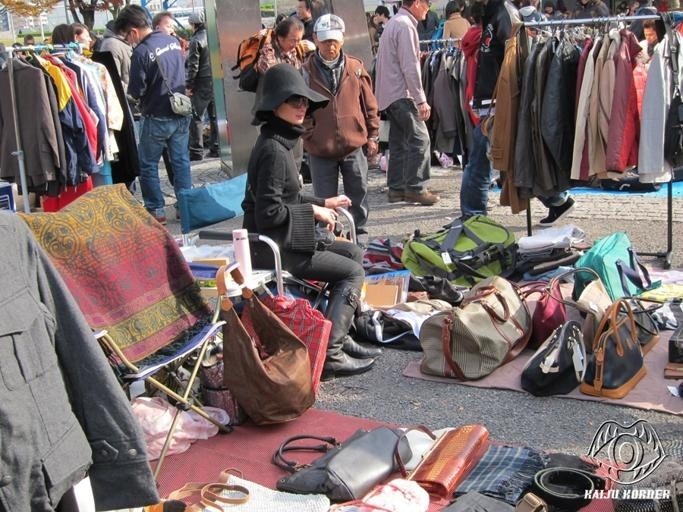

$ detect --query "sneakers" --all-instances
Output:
[539,196,574,226]
[190,157,202,161]
[157,217,167,224]
[206,152,218,157]
[175,210,180,222]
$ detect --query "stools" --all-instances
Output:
[250,204,357,310]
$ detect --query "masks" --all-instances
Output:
[129,32,137,50]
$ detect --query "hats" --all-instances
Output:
[188,7,205,23]
[250,62,330,125]
[313,13,345,41]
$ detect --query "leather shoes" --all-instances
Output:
[388,189,405,203]
[404,189,440,205]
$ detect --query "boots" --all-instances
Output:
[320,280,375,380]
[343,333,382,358]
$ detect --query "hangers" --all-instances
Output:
[416,37,466,52]
[3,41,82,60]
[520,11,643,38]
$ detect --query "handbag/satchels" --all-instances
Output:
[521,320,587,397]
[400,212,519,289]
[218,262,315,426]
[336,477,430,512]
[572,230,662,307]
[272,426,413,503]
[579,299,647,399]
[409,273,469,305]
[142,42,192,116]
[605,295,664,356]
[544,266,613,355]
[354,310,423,351]
[515,279,566,349]
[192,468,330,512]
[419,275,533,382]
[481,78,498,136]
[396,423,489,499]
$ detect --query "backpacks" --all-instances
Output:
[230,29,271,79]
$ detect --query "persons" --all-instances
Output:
[257,17,313,76]
[186,11,221,160]
[151,11,187,52]
[242,64,382,366]
[2,20,130,98]
[114,4,194,225]
[365,6,391,41]
[296,0,316,39]
[418,0,682,60]
[300,14,380,238]
[375,0,440,205]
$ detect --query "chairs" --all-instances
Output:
[17,180,233,488]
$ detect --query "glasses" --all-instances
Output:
[124,31,130,41]
[425,0,432,7]
[284,95,308,106]
